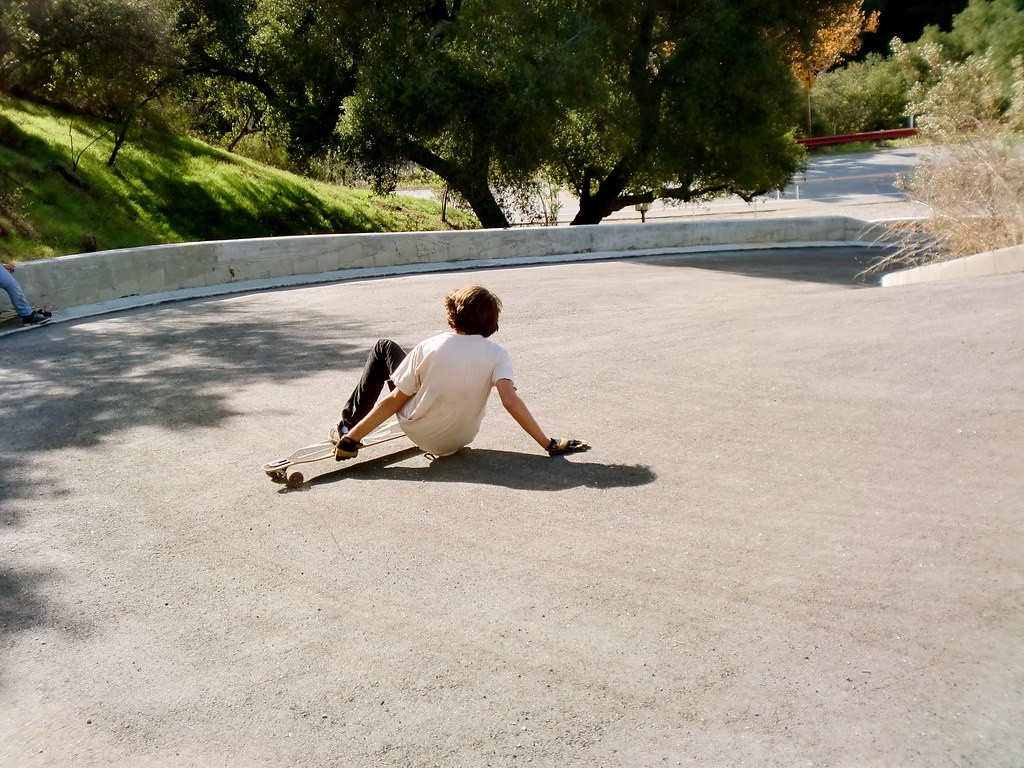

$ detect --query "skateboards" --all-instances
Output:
[260,421,406,489]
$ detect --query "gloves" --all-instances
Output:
[331,435,363,462]
[545,437,588,455]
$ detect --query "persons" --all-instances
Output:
[328,286,589,462]
[0,263,53,326]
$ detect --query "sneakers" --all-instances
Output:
[328,420,360,447]
[22,311,51,326]
